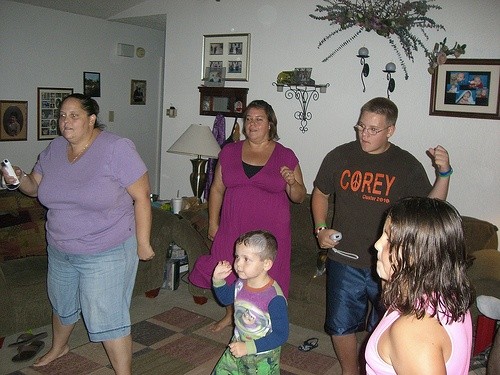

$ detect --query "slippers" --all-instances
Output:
[8,332,48,347]
[12,341,45,362]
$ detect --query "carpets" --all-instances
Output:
[8,306,343,375]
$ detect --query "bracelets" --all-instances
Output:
[314,224,327,234]
[436,165,453,177]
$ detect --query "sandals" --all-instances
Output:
[298,338,319,352]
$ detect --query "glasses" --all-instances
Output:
[354,124,394,135]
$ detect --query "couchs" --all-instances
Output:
[0,206,178,338]
[172,194,500,370]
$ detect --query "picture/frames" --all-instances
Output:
[0,100,28,141]
[130,80,147,106]
[429,59,500,120]
[83,72,100,97]
[37,87,73,140]
[201,33,250,81]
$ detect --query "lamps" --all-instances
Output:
[167,124,221,203]
[382,62,396,98]
[355,46,369,92]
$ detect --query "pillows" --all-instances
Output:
[0,190,48,261]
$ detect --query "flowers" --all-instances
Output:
[309,0,466,80]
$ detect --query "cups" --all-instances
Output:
[172,199,182,214]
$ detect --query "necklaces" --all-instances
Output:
[70,138,92,165]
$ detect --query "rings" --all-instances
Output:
[288,172,290,175]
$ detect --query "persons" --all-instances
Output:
[448,72,488,104]
[135,85,143,102]
[211,231,289,375]
[365,196,473,375]
[310,97,453,375]
[8,112,20,135]
[210,43,243,73]
[0,93,155,375]
[188,100,307,333]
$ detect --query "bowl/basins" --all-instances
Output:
[150,193,159,202]
